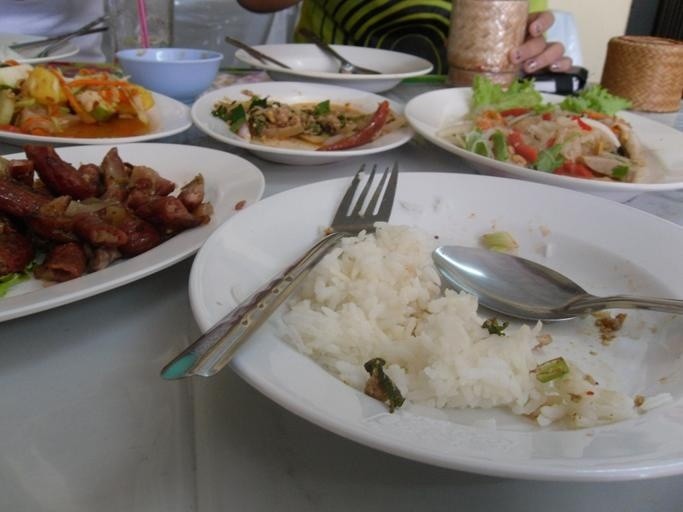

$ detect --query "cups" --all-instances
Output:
[446,0,526,85]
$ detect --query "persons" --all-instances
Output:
[233,1,577,81]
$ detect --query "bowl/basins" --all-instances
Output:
[116,47,224,105]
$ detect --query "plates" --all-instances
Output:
[0,143,266,323]
[404,86,683,205]
[234,45,434,94]
[0,88,195,142]
[188,80,415,166]
[1,31,82,64]
[187,167,682,484]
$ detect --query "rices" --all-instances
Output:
[282,221,673,428]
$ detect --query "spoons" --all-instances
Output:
[299,25,383,74]
[430,243,682,323]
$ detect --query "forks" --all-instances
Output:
[158,162,398,382]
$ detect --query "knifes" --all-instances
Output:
[10,15,110,57]
